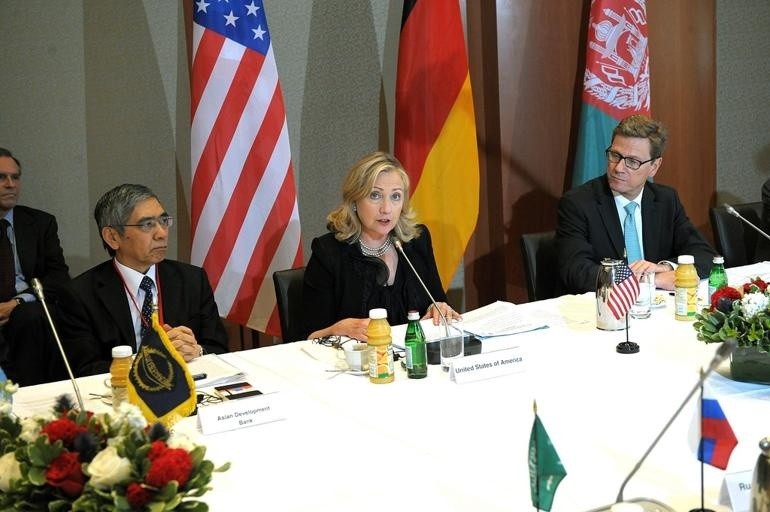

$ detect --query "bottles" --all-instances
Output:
[750,438,769,511]
[404,311,428,379]
[366,308,396,384]
[674,254,699,321]
[594,257,632,332]
[110,345,139,415]
[707,254,728,306]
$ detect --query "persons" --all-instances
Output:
[46,184,228,383]
[544,113,717,295]
[0,147,71,354]
[754,178,769,262]
[296,150,463,343]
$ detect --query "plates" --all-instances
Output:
[341,368,368,377]
[651,292,670,309]
[101,391,114,406]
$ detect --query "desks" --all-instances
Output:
[1,259,770,512]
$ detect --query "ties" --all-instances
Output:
[0,216,18,305]
[139,276,154,342]
[623,201,642,265]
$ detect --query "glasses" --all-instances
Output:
[109,215,175,233]
[604,145,659,170]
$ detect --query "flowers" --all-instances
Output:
[0,374,234,512]
[694,276,770,354]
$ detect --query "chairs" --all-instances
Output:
[711,200,770,268]
[274,268,307,341]
[522,230,557,303]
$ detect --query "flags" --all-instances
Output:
[572,0,650,187]
[128,310,198,429]
[527,416,566,512]
[183,1,303,344]
[608,264,641,320]
[697,403,737,469]
[394,0,480,294]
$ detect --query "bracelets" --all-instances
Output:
[16,298,23,305]
[662,261,670,265]
[199,344,205,356]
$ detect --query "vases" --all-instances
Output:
[729,346,770,386]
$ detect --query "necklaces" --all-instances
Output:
[359,235,392,257]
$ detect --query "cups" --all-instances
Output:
[631,271,653,319]
[342,342,368,372]
[438,316,465,372]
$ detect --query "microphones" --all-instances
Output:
[721,203,770,240]
[584,337,738,512]
[31,277,87,412]
[391,237,481,364]
